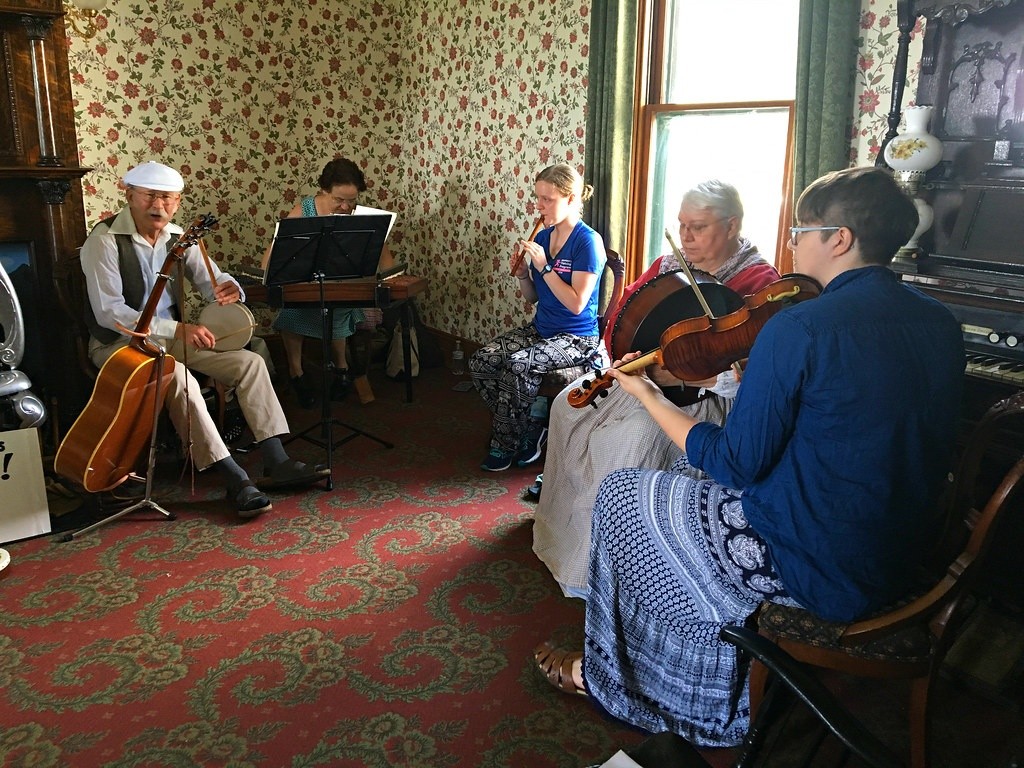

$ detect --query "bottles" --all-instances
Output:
[452,341,471,371]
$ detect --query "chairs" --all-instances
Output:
[749,392,1024,768]
[46,251,220,490]
[596,624,906,768]
[546,246,628,402]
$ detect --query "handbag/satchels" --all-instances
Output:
[385,320,419,382]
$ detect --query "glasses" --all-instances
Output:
[789,224,854,246]
[679,216,730,236]
[333,198,357,206]
[131,188,179,205]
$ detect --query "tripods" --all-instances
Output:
[64,338,178,543]
[256,214,395,492]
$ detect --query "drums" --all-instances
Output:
[196,298,257,352]
[608,269,747,409]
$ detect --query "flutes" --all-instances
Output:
[509,213,544,278]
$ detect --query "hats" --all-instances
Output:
[124,161,184,192]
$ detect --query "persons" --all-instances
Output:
[261,157,395,410]
[534,163,969,750]
[80,161,331,515]
[467,164,608,474]
[533,178,781,601]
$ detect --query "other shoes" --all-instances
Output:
[528,474,544,498]
[290,372,321,412]
[329,365,352,400]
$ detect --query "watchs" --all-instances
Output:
[539,263,553,277]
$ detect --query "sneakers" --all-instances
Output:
[480,440,520,472]
[518,425,549,468]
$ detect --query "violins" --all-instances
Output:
[566,277,823,411]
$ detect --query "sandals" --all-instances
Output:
[222,479,273,518]
[531,638,613,722]
[272,457,331,489]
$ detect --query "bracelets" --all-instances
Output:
[516,269,529,280]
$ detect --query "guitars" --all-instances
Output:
[54,209,222,495]
